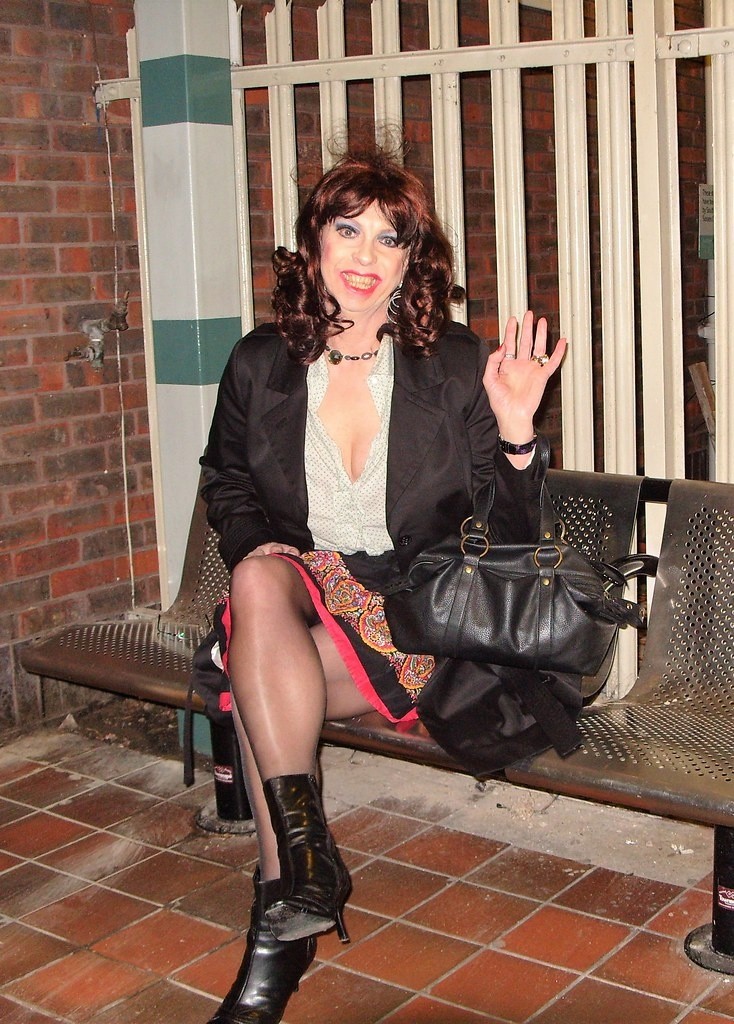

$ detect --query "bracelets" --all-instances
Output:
[498,434,538,455]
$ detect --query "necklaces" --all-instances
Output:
[324,345,379,365]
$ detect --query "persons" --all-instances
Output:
[198,129,567,1024]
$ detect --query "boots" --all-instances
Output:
[209,867,317,1023]
[252,772,353,945]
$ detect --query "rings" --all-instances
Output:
[531,354,550,366]
[504,354,515,358]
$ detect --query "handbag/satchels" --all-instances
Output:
[380,427,644,676]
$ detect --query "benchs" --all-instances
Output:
[20,445,734,975]
